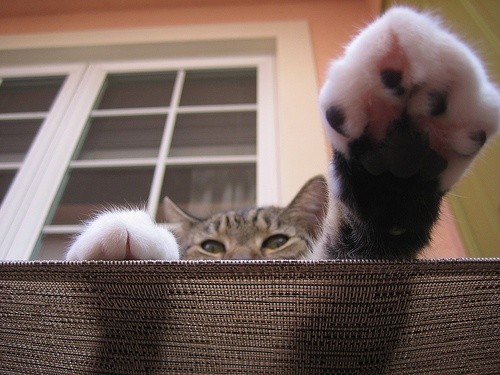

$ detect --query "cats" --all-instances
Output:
[65,5,500,375]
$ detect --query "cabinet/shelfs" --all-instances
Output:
[1,1,329,262]
